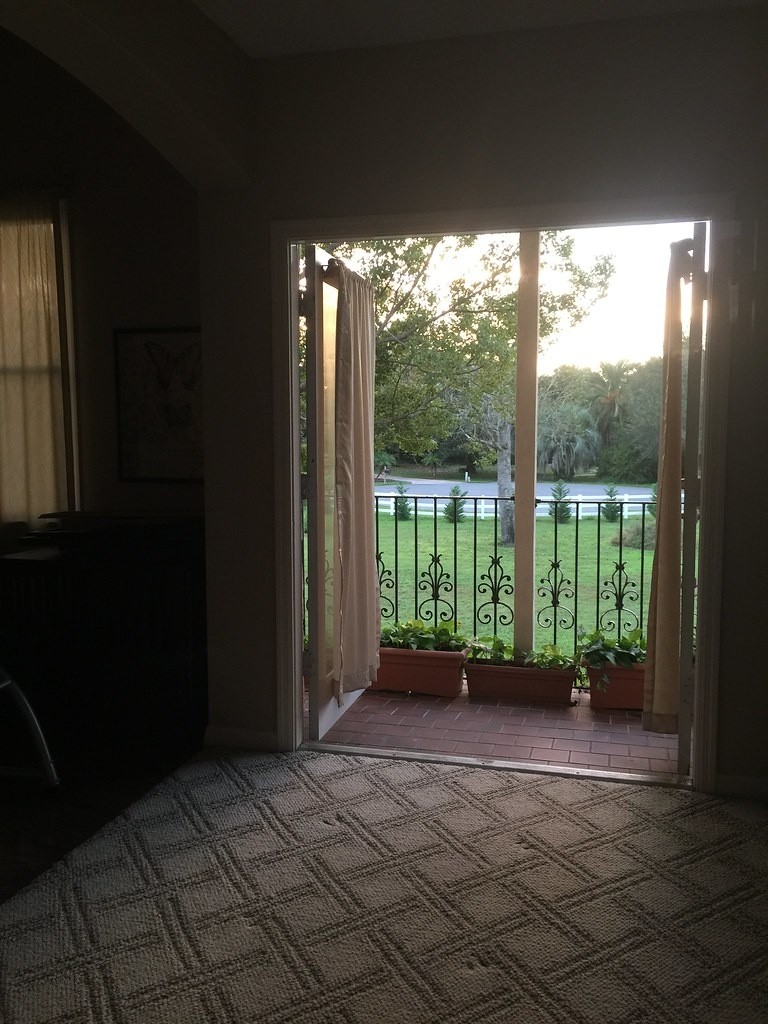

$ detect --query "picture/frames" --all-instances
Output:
[113,327,202,485]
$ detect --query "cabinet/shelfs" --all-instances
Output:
[0,526,207,766]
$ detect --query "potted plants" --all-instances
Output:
[366,621,649,711]
